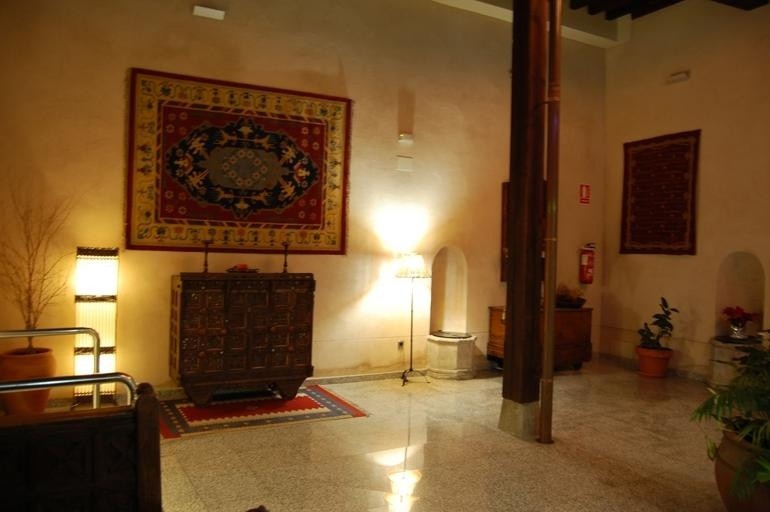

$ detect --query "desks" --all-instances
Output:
[489,303,593,376]
[165,272,317,409]
[0,385,169,510]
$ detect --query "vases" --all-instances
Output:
[729,319,745,341]
[1,347,57,417]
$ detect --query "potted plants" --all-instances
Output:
[634,294,681,379]
[687,335,769,512]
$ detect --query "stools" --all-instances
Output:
[428,330,478,381]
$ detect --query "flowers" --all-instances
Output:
[722,302,760,323]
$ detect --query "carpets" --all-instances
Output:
[151,384,371,441]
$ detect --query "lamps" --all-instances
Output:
[69,247,122,409]
[395,252,432,388]
